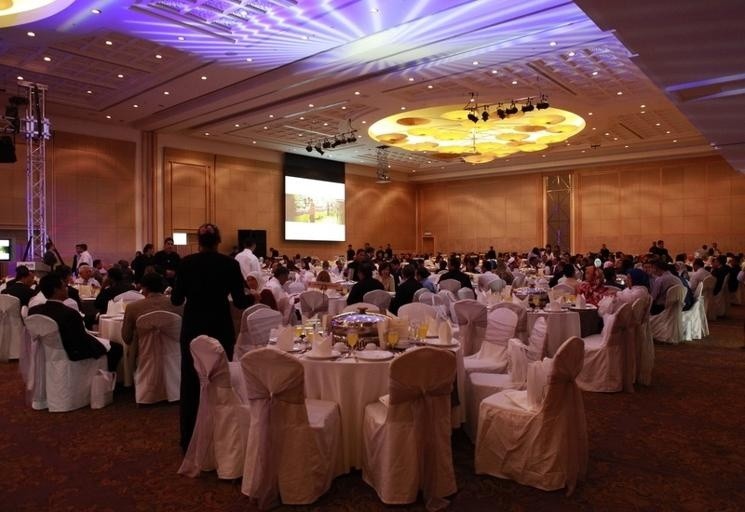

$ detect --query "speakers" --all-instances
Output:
[0,135,17,164]
[239,230,266,263]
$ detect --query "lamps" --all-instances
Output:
[306,118,358,155]
[462,77,550,123]
[367,104,586,165]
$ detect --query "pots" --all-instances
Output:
[328,305,393,345]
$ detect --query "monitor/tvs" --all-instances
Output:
[0,239,12,261]
[172,232,187,245]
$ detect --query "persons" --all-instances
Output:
[171,223,262,457]
[4,238,741,394]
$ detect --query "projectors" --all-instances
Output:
[375,175,391,184]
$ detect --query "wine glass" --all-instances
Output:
[292,323,314,347]
[386,330,399,352]
[345,326,359,352]
[416,323,428,344]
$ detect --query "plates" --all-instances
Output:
[304,349,342,360]
[484,304,596,313]
[99,311,125,321]
[354,349,396,361]
[281,344,303,352]
[423,331,457,347]
